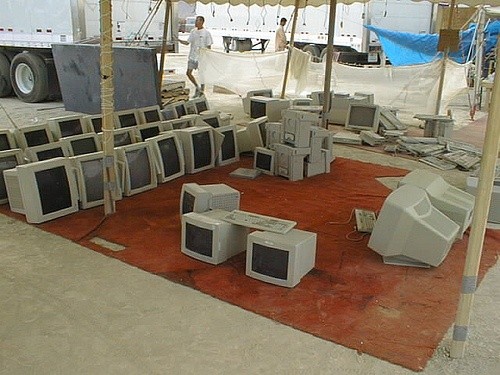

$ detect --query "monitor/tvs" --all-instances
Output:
[2,157,79,224]
[237,89,374,181]
[180,182,240,214]
[345,103,381,133]
[367,168,500,268]
[181,209,249,265]
[0,96,239,210]
[246,228,317,288]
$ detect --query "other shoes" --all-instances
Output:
[193,88,205,99]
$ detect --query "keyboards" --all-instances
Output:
[223,211,298,233]
[355,208,376,233]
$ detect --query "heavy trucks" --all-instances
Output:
[195,0,500,87]
[0,0,180,105]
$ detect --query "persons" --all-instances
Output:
[173,16,212,99]
[275,18,289,53]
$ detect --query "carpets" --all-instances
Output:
[0,152,500,370]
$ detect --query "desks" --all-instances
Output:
[415,113,456,139]
